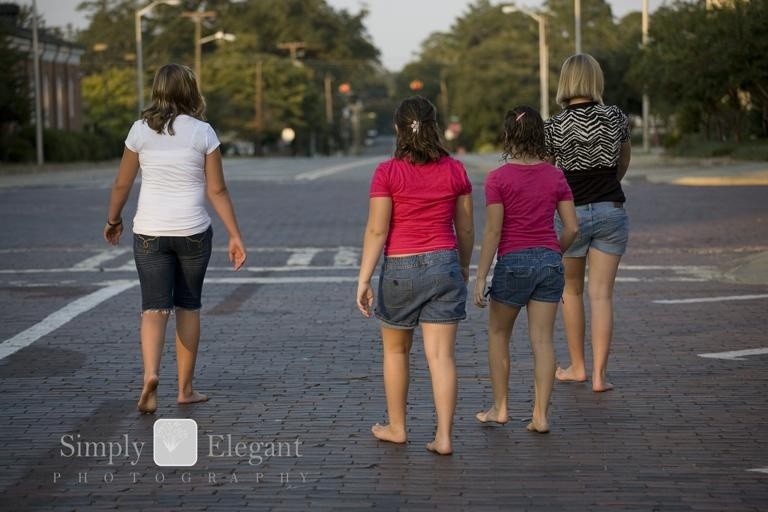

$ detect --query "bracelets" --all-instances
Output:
[106,219,124,227]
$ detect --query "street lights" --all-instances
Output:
[134,0,182,115]
[500,3,551,124]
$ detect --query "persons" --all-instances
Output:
[473,102,581,434]
[536,51,633,394]
[102,61,248,415]
[355,94,476,456]
